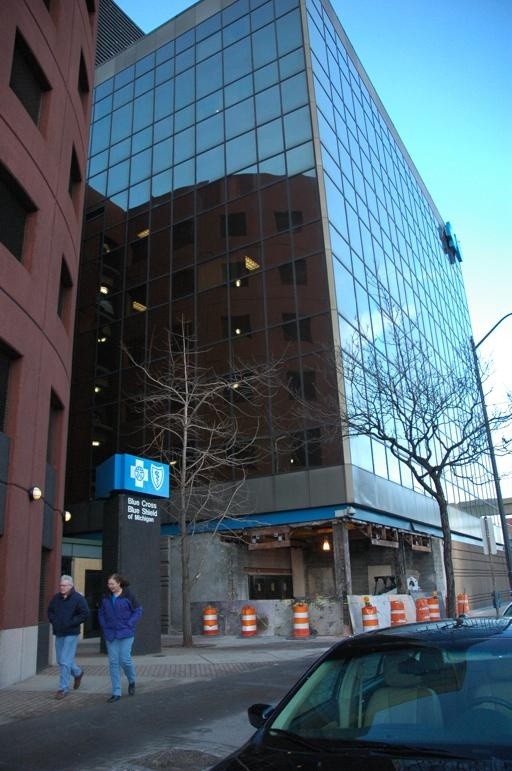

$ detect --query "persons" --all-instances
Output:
[47,574,90,701]
[98,574,144,702]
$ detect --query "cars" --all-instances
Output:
[209,616,510,769]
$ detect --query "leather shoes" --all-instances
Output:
[56,691,64,700]
[106,695,121,703]
[129,682,135,695]
[74,671,84,689]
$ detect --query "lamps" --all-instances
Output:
[63,510,72,522]
[29,486,42,500]
[323,535,331,551]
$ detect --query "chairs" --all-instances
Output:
[471,656,512,718]
[420,648,466,694]
[363,650,446,733]
